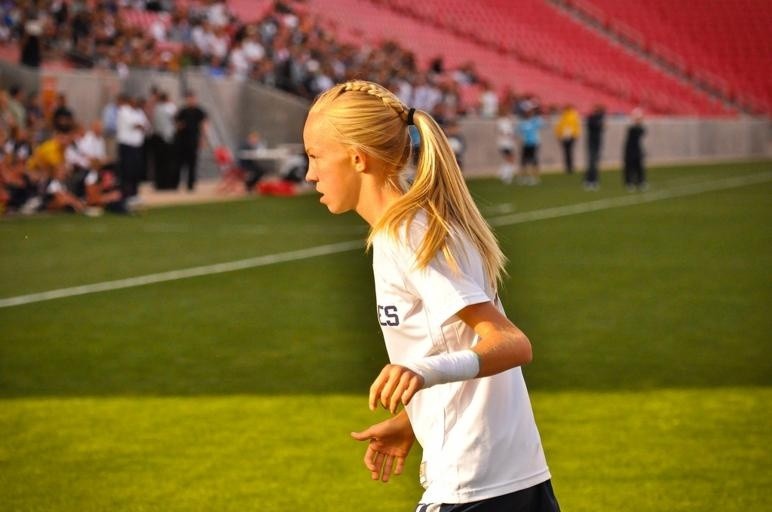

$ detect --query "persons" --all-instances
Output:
[286,13,444,111]
[0,1,309,217]
[433,60,548,184]
[304,80,559,512]
[555,101,645,191]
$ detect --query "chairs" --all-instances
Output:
[201,117,246,194]
